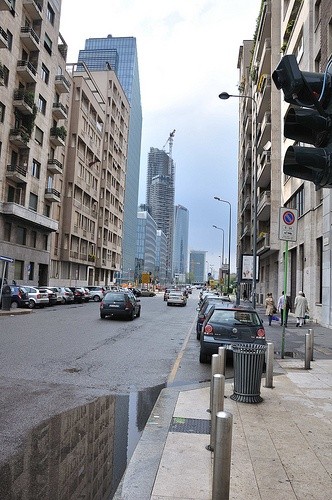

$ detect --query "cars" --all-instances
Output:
[0,283,157,309]
[198,302,266,363]
[164,281,237,340]
[100,289,141,321]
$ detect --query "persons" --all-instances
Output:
[243,290,247,302]
[265,293,275,326]
[295,291,308,328]
[277,290,289,328]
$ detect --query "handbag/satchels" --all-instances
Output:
[303,315,309,319]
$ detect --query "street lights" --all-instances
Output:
[214,196,232,292]
[218,92,257,310]
[213,225,225,296]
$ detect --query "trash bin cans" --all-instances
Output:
[228,342,268,405]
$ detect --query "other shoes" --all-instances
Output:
[296,323,299,327]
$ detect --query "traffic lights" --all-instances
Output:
[270,54,332,191]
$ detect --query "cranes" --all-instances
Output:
[162,129,177,182]
